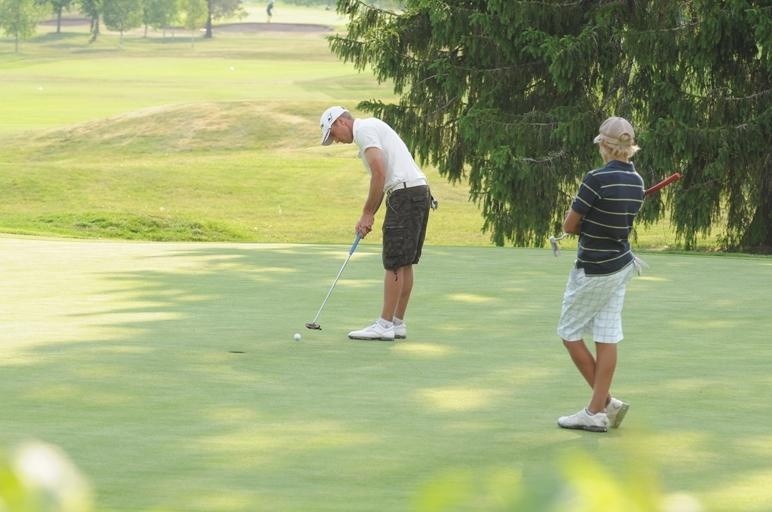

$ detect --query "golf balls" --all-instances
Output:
[294,334,300,341]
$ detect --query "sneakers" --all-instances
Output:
[395,324,407,339]
[347,320,396,341]
[605,397,630,429]
[558,408,610,432]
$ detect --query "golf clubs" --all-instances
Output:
[549,172,679,257]
[305,234,362,329]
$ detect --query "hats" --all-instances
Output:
[318,105,349,147]
[593,116,635,145]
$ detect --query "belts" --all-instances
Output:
[384,179,429,195]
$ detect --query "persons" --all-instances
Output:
[319,105,431,340]
[557,116,648,432]
